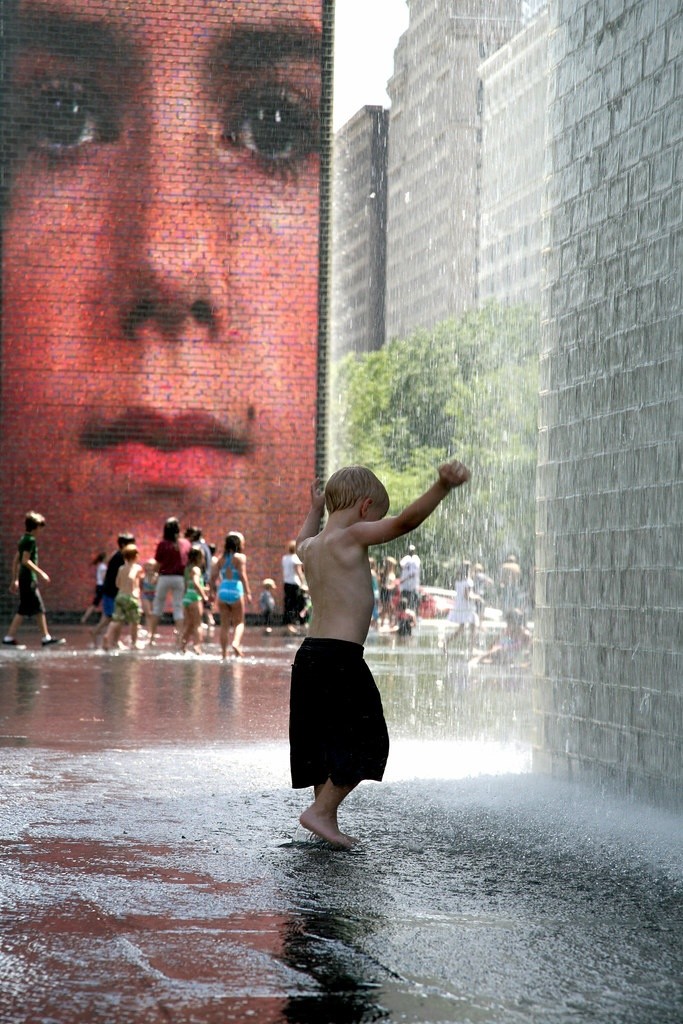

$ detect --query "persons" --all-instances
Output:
[281,538,310,634]
[256,577,278,633]
[442,553,535,669]
[75,517,263,667]
[0,21,319,614]
[361,543,426,641]
[288,459,471,851]
[0,510,66,651]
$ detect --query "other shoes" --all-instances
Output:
[2,640,27,649]
[42,638,66,648]
